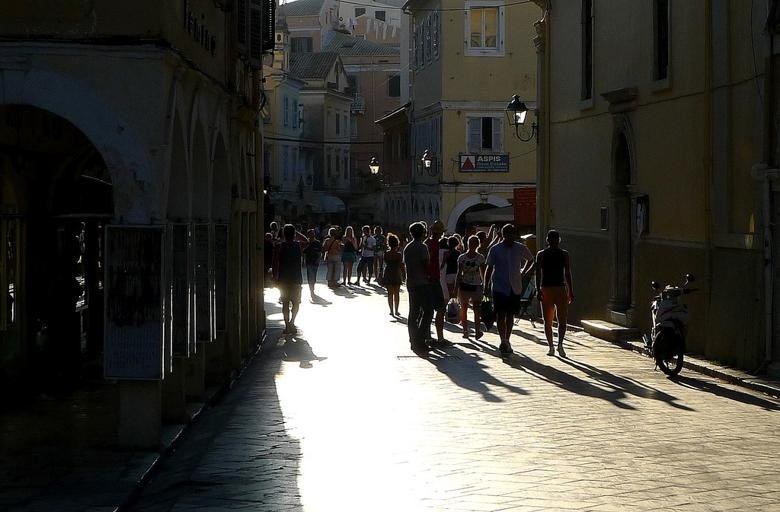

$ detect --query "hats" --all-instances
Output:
[430,220,447,232]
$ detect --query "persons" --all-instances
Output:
[402,221,573,356]
[383,233,405,316]
[266,222,387,334]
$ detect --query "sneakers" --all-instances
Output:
[475,332,483,340]
[547,350,555,356]
[557,347,566,357]
[500,342,513,354]
[411,337,452,358]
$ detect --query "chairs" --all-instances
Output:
[515,288,536,328]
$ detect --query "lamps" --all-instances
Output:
[503,94,539,144]
[368,153,385,184]
[421,147,438,177]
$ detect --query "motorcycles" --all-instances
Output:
[641,272,696,376]
